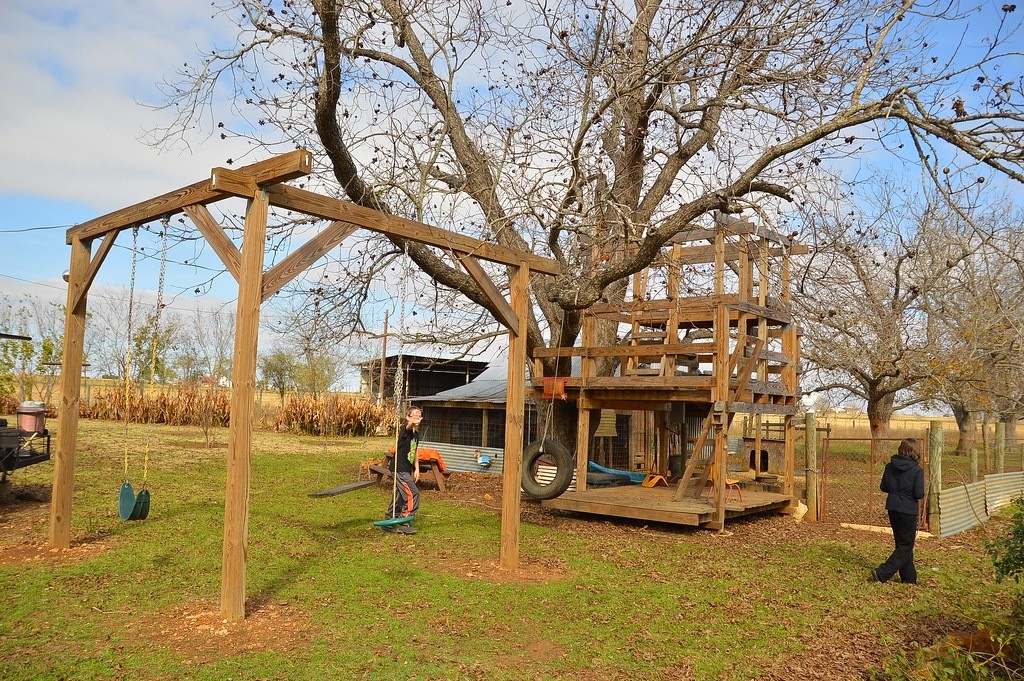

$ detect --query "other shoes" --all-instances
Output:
[381,525,396,533]
[871,569,881,582]
[397,523,418,533]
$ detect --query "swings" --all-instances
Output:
[305,231,381,501]
[113,212,172,522]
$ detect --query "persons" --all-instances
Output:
[381,405,423,534]
[871,438,926,586]
